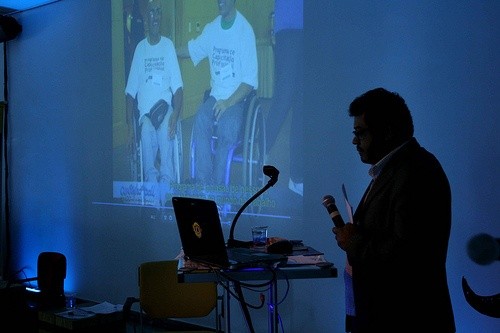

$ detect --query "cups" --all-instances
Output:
[65,293,77,310]
[250,225,269,248]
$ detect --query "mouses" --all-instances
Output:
[267,240,294,254]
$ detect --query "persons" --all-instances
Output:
[332,88,455,333]
[126,1,183,223]
[175,0,259,218]
[264,1,303,199]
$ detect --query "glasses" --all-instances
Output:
[353,128,372,138]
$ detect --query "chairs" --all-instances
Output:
[4,252,66,333]
[121,262,218,333]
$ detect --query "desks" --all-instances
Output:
[179,239,338,333]
[0,297,227,333]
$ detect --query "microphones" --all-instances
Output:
[321,195,346,228]
[227,165,280,246]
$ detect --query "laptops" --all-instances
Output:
[172,197,288,270]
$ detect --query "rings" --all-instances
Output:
[340,241,343,244]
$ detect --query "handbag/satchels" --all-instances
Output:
[148,99,169,129]
